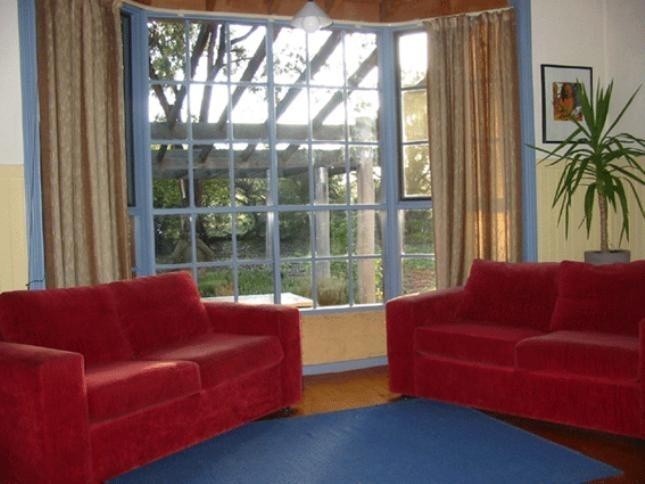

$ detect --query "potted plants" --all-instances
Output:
[523,73,645,266]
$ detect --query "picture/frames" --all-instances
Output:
[539,62,598,145]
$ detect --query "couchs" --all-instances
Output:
[0,266,307,484]
[384,257,643,440]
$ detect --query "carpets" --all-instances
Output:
[106,394,628,483]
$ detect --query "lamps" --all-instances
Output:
[291,0,332,34]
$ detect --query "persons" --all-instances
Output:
[557,83,575,117]
